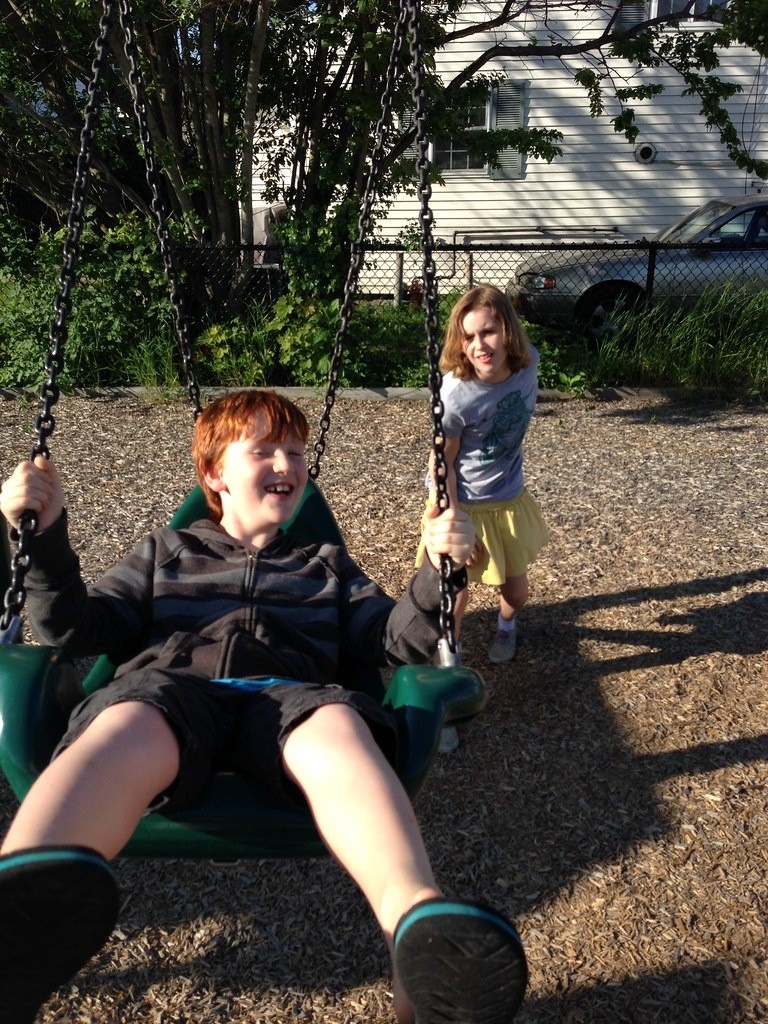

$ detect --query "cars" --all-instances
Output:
[507,193,768,320]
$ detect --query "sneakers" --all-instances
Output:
[438,726,459,751]
[487,625,516,662]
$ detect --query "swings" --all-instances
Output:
[0,0,487,855]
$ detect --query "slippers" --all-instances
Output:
[390,897,527,1023]
[0,845,121,1024]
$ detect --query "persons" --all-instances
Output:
[0,388,529,1022]
[414,283,550,757]
